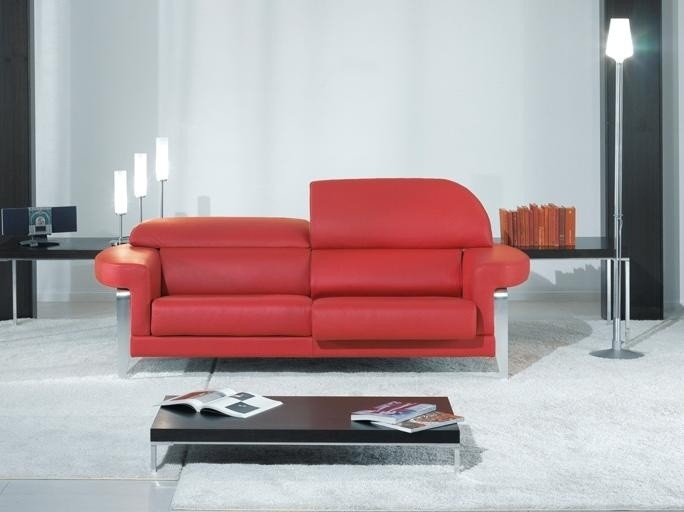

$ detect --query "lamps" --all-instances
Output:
[109,135,169,248]
[591,11,639,360]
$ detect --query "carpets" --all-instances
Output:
[0,317,217,475]
[170,315,681,507]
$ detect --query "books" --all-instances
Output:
[499,203,577,247]
[153,388,284,419]
[370,411,464,433]
[351,401,438,425]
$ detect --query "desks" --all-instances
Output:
[492,236,635,330]
[2,237,128,323]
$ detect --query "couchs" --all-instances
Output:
[93,176,534,382]
